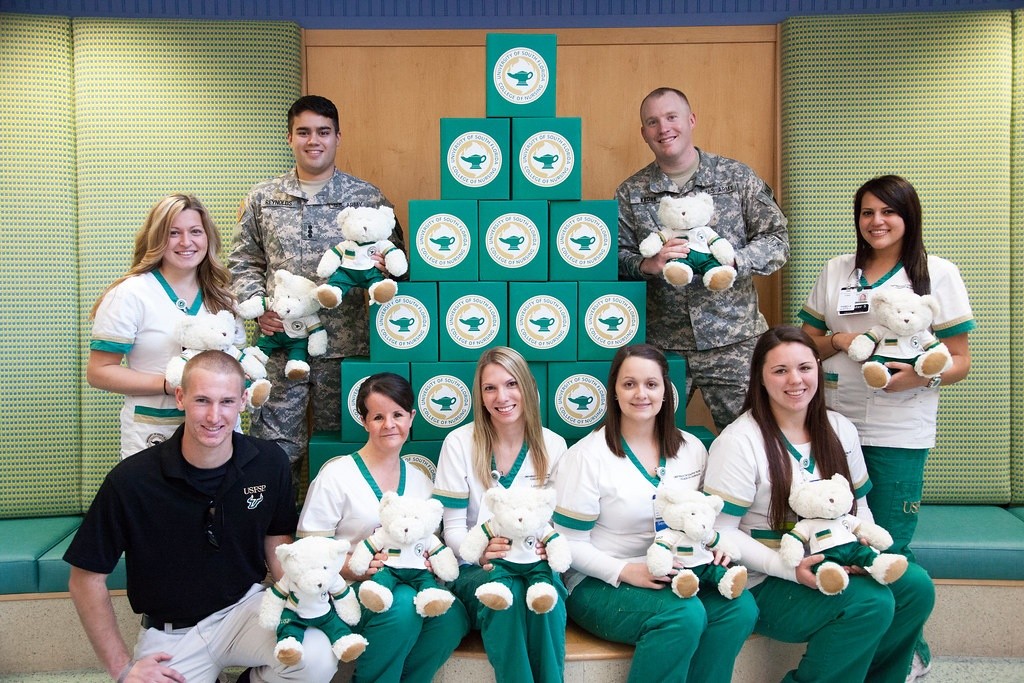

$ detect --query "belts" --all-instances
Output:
[141,613,197,631]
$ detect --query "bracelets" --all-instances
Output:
[163,379,169,396]
[117,658,137,683]
[831,332,843,352]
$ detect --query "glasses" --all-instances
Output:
[204,495,227,553]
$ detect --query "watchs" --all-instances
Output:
[925,376,941,389]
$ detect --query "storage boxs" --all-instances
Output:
[309,34,716,489]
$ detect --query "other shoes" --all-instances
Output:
[904,651,931,683]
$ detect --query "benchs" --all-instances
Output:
[0,503,1024,683]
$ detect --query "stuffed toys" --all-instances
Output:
[165,207,409,412]
[647,487,747,600]
[779,473,908,596]
[638,193,737,292]
[846,287,953,391]
[261,486,571,666]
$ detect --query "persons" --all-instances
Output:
[550,344,759,683]
[703,326,936,683]
[86,194,242,458]
[63,350,339,683]
[611,88,790,435]
[859,294,866,300]
[797,175,977,683]
[227,95,407,507]
[296,373,472,683]
[431,346,568,683]
